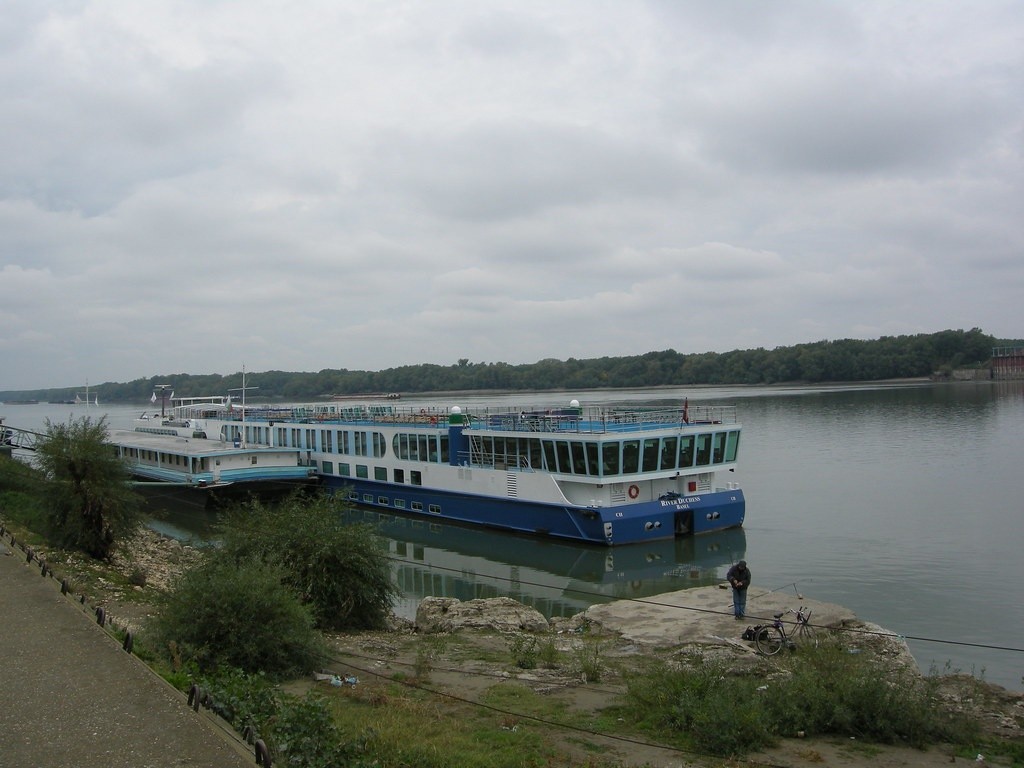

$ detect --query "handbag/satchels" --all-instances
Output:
[742,625,768,640]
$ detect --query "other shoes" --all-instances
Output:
[735,616,743,620]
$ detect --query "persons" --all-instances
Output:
[727,561,751,620]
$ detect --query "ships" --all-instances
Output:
[134,361,746,548]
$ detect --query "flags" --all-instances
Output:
[682,400,688,425]
[150,392,157,403]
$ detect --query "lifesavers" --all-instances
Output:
[628,483,639,499]
[421,409,425,413]
[318,415,323,420]
